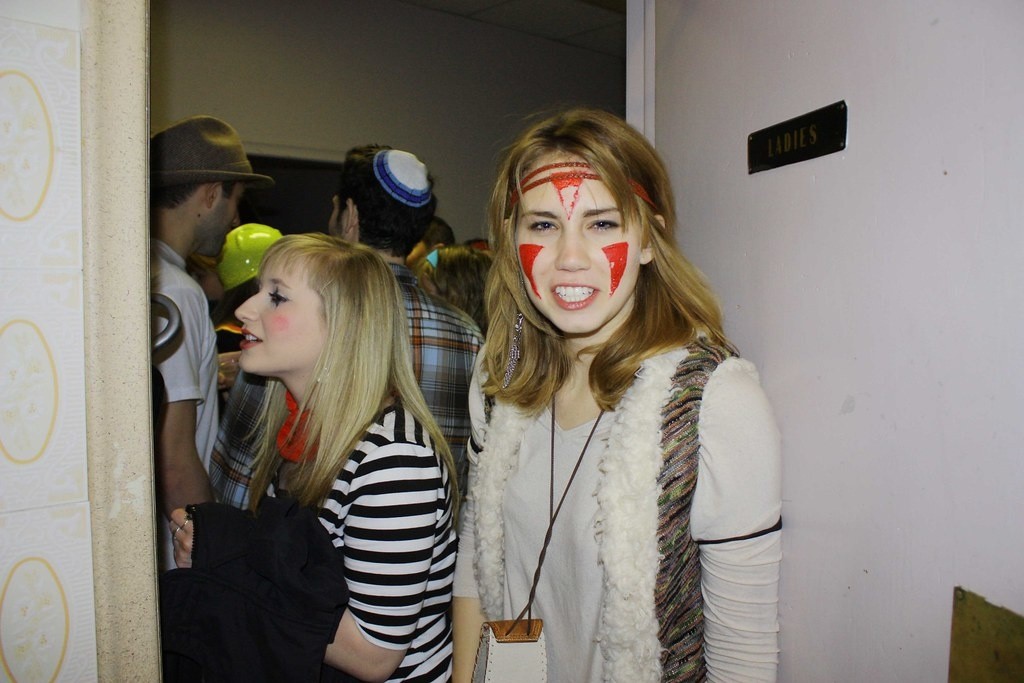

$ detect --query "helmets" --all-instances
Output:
[217,224,285,293]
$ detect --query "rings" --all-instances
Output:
[171,537,177,544]
[173,526,182,535]
[180,519,189,529]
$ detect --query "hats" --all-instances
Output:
[150,115,275,188]
[374,148,433,207]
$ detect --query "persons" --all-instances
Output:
[450,107,783,683]
[169,233,462,682]
[148,116,493,570]
